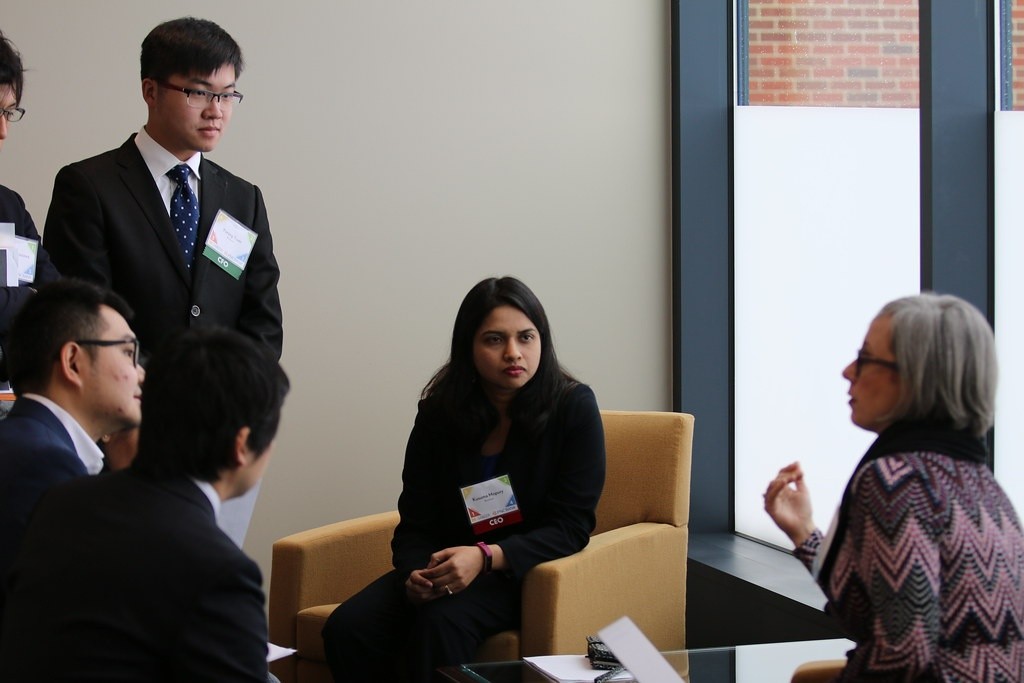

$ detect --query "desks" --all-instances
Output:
[437,636,857,683]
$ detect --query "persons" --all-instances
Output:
[0,28,66,382]
[0,276,147,572]
[763,292,1024,683]
[42,18,283,474]
[0,323,290,683]
[320,276,606,683]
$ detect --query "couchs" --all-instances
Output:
[268,411,697,683]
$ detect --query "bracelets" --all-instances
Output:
[475,542,493,576]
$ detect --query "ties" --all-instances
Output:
[165,164,200,277]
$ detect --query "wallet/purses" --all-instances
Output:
[584,633,629,682]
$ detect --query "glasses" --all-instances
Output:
[855,349,899,377]
[0,108,25,122]
[159,83,243,109]
[75,338,140,369]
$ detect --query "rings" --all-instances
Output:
[444,584,454,598]
